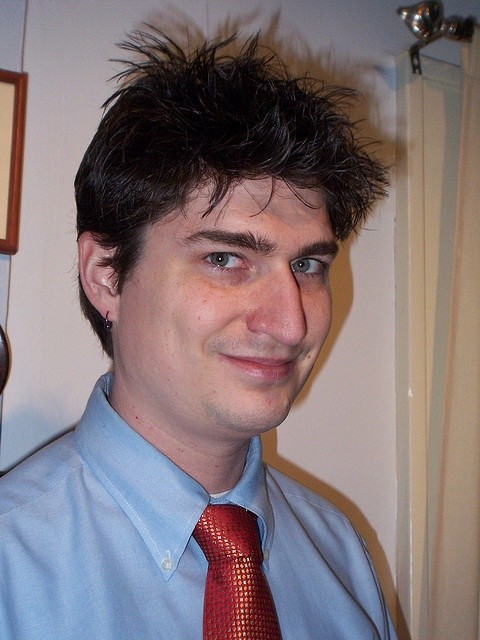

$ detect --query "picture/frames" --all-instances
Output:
[1,69,28,256]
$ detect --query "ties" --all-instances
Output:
[190,503,284,640]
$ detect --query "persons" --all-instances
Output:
[1,20,398,639]
